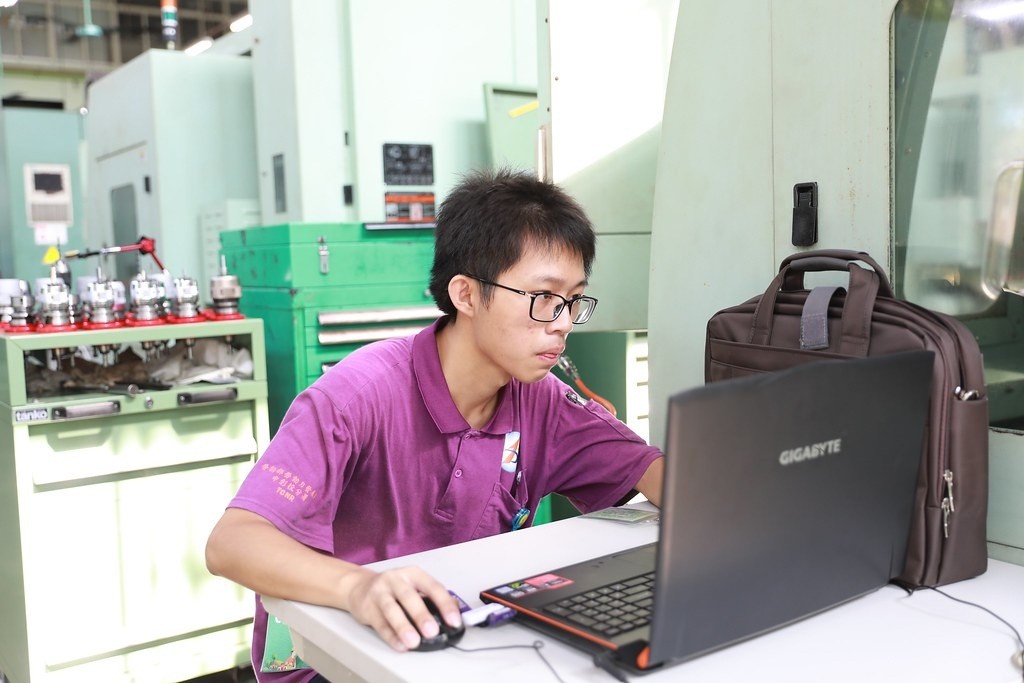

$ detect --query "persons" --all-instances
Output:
[205,172,666,683]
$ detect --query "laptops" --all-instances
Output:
[479,350,936,675]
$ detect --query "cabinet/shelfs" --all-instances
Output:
[0,319,274,683]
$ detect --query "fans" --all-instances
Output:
[7,0,162,45]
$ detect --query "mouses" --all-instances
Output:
[396,597,466,653]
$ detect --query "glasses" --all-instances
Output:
[457,272,599,324]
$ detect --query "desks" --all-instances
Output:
[259,499,1023,682]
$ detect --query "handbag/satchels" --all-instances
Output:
[704,248,989,589]
